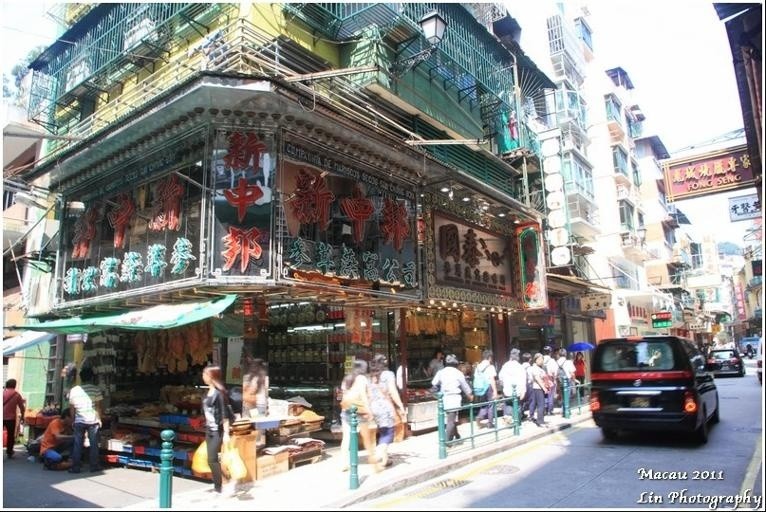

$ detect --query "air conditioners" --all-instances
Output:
[124,18,158,62]
[65,58,96,98]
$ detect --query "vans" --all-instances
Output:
[584,333,722,447]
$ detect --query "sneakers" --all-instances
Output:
[28,456,105,474]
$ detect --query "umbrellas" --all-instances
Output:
[567,342,595,352]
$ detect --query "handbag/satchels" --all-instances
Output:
[243,384,258,403]
[213,393,236,425]
[338,395,370,415]
[219,448,245,483]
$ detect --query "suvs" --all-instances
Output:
[708,347,747,379]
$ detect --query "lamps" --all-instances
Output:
[389,10,448,79]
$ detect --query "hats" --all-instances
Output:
[445,345,567,366]
[350,359,366,370]
[249,358,266,368]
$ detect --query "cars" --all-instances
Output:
[709,336,760,359]
[756,355,762,387]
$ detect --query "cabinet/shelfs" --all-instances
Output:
[267,300,343,440]
[92,329,207,477]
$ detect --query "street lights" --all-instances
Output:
[10,191,86,411]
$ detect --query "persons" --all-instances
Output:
[3,345,588,492]
